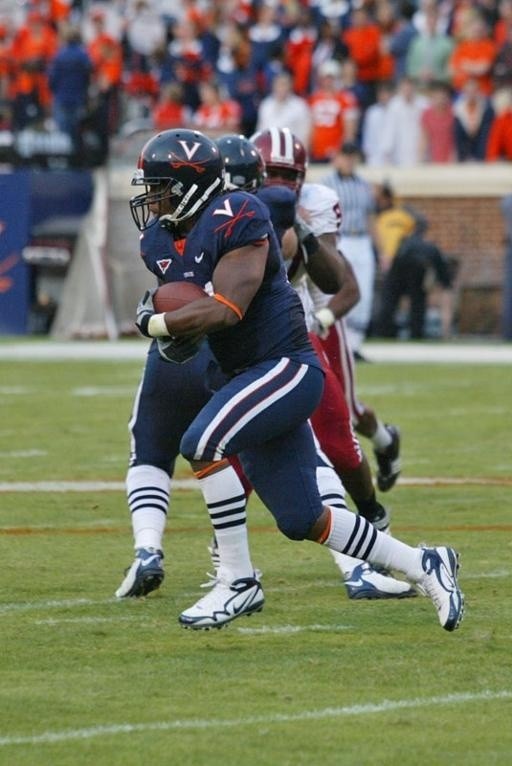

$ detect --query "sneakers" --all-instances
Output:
[179,568,265,631]
[358,502,392,536]
[373,422,401,492]
[407,542,466,632]
[343,561,418,600]
[115,547,166,596]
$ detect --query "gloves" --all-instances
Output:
[155,332,204,366]
[133,286,158,339]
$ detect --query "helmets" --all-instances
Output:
[128,127,226,232]
[250,125,307,200]
[215,133,265,195]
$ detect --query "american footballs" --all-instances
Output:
[153,281,210,313]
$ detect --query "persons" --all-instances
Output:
[113,132,417,601]
[210,206,394,579]
[248,128,400,491]
[0,0,512,362]
[128,127,467,631]
